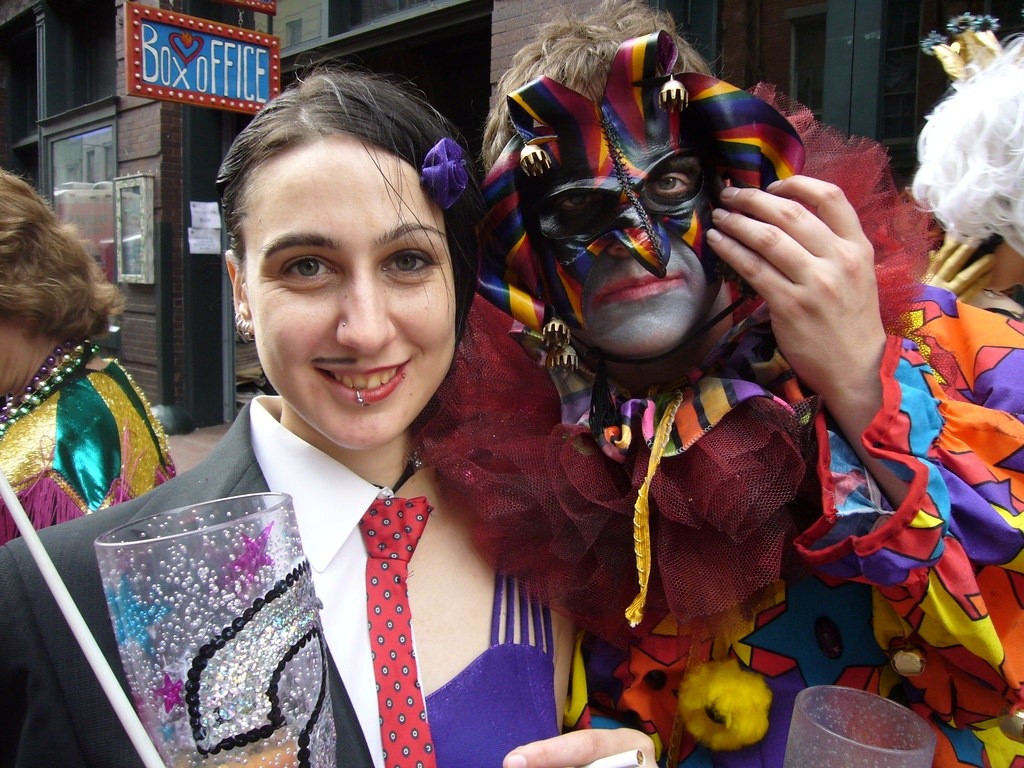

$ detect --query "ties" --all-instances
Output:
[358,495,437,768]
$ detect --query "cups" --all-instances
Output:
[93,493,336,768]
[782,684,937,768]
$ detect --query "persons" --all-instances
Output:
[0,74,658,768]
[480,0,1024,768]
[0,169,177,546]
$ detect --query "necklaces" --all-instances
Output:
[0,338,101,437]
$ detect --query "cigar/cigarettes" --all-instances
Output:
[581,749,645,768]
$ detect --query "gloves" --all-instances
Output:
[918,236,996,305]
